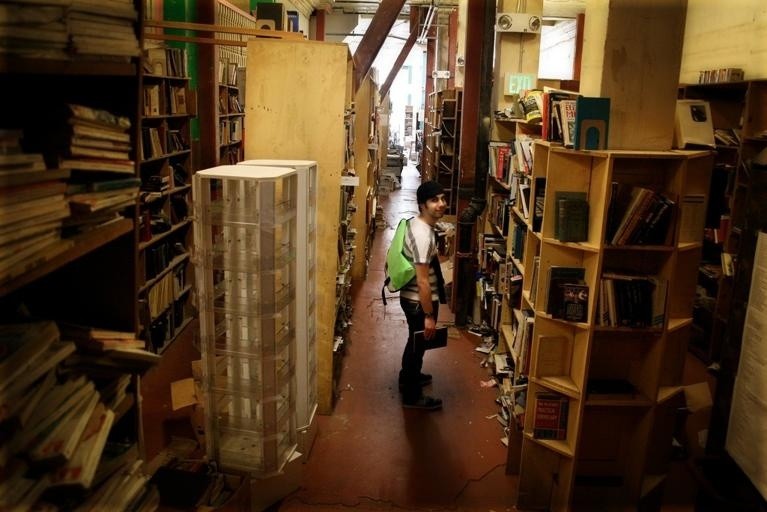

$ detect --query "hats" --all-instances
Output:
[416,181,443,204]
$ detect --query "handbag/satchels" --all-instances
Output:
[383,217,416,293]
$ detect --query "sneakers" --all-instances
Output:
[401,396,443,410]
[418,373,432,385]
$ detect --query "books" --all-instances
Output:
[692,216,738,307]
[141,84,187,161]
[603,269,668,331]
[256,2,299,39]
[412,326,447,354]
[1,321,234,512]
[218,60,243,164]
[714,128,743,146]
[468,86,611,431]
[696,67,745,84]
[138,163,191,355]
[532,392,568,441]
[1,105,142,272]
[147,48,188,78]
[1,1,141,65]
[608,181,677,246]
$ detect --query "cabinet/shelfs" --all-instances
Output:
[418,2,766,512]
[2,0,392,512]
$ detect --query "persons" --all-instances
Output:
[397,181,449,410]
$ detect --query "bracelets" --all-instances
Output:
[423,312,434,318]
